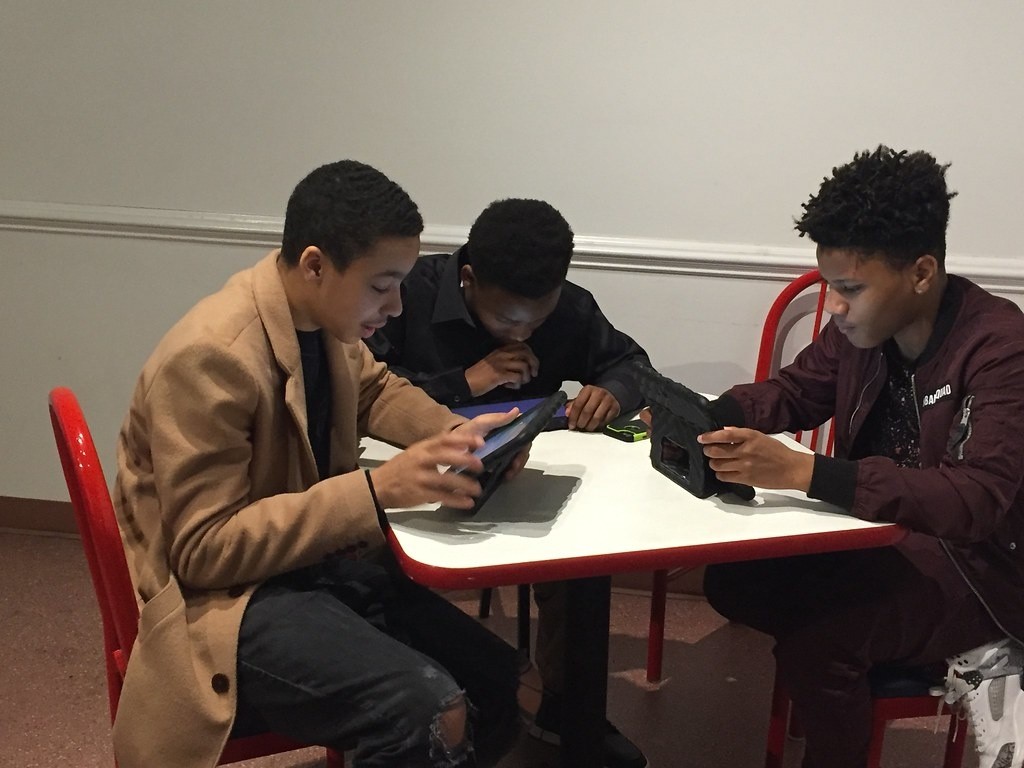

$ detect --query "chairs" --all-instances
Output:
[638,264,971,768]
[46,385,345,768]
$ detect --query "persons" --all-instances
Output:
[110,161,543,768]
[361,199,659,768]
[641,152,1024,768]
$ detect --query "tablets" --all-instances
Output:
[448,394,570,433]
[428,391,568,503]
[632,362,756,502]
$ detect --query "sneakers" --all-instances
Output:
[932,636,1023,767]
[529,705,651,768]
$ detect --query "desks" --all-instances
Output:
[355,392,908,768]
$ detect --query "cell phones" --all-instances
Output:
[604,419,650,442]
